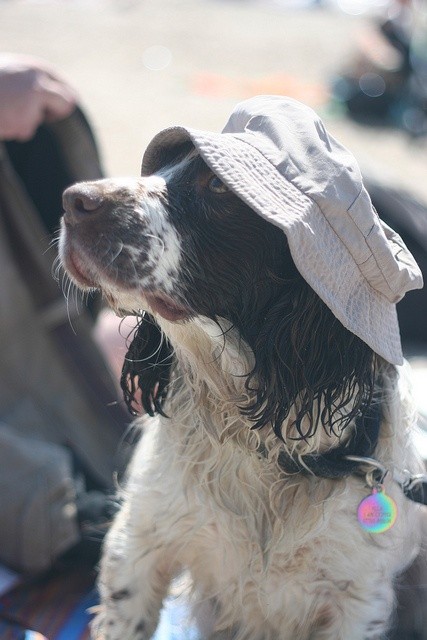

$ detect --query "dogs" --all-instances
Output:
[41,95,426,640]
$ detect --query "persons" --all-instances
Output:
[0,54,146,576]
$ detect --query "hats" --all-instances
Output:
[141,93,423,367]
